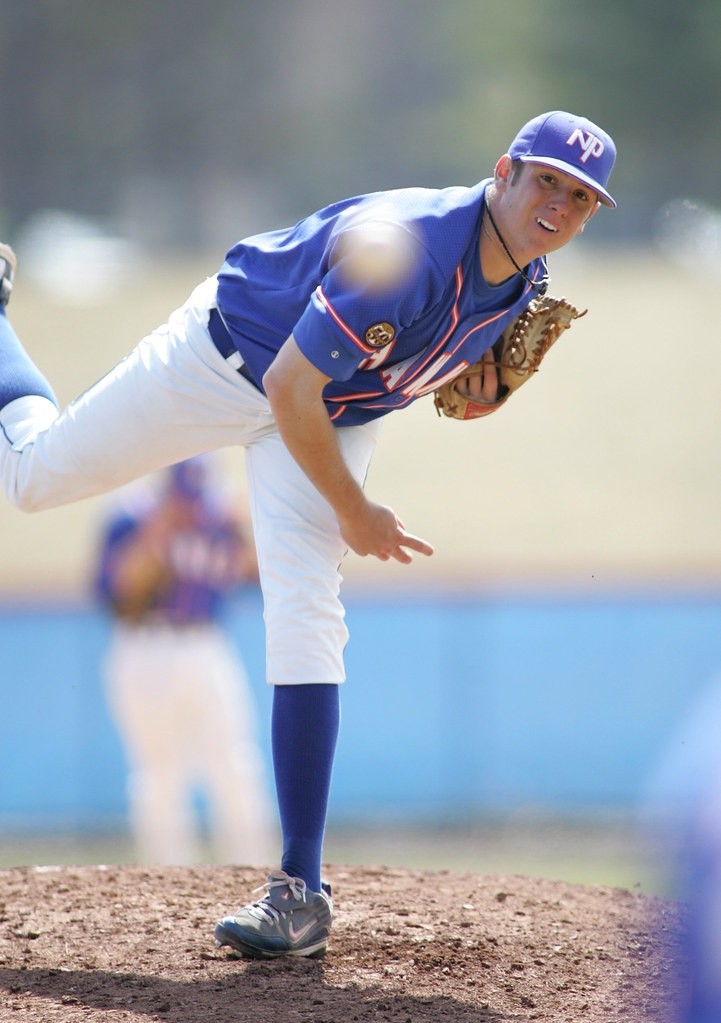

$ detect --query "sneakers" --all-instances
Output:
[213,869,333,957]
[0,243,16,304]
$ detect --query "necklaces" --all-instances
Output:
[482,187,551,295]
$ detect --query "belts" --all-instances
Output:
[209,309,265,394]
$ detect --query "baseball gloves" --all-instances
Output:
[436,296,587,421]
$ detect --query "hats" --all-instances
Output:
[508,110,618,208]
[174,459,222,526]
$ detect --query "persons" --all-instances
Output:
[0,109,618,960]
